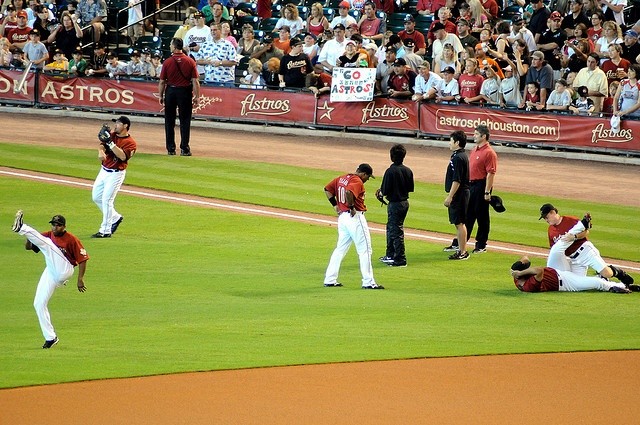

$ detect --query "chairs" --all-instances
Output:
[160,24,180,37]
[377,11,387,20]
[114,47,130,61]
[253,29,266,43]
[349,9,361,21]
[322,7,336,22]
[159,37,171,49]
[236,2,257,15]
[272,4,284,17]
[267,31,280,42]
[160,50,172,63]
[133,35,154,49]
[297,5,310,19]
[298,33,305,41]
[408,0,419,17]
[415,26,428,39]
[415,14,434,28]
[262,17,279,31]
[387,25,405,35]
[389,12,410,26]
[237,14,261,30]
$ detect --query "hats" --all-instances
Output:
[538,203,557,220]
[346,23,358,30]
[554,77,568,86]
[455,19,468,25]
[279,25,290,32]
[568,0,583,4]
[390,58,406,66]
[152,51,161,58]
[49,215,66,225]
[141,45,151,54]
[440,66,455,73]
[459,2,469,10]
[29,28,41,35]
[385,45,396,52]
[130,48,141,57]
[17,11,27,19]
[358,163,376,179]
[502,64,516,71]
[289,36,305,45]
[610,114,621,133]
[194,9,205,16]
[333,23,345,30]
[550,10,561,20]
[112,116,130,126]
[624,29,638,38]
[511,261,531,271]
[12,47,23,54]
[484,65,498,72]
[403,13,414,22]
[345,39,356,46]
[338,0,350,8]
[577,85,589,98]
[403,37,414,47]
[490,196,506,212]
[304,32,317,41]
[92,41,105,50]
[7,3,16,11]
[106,50,118,58]
[53,48,64,55]
[263,35,274,43]
[417,60,430,70]
[512,14,524,24]
[530,50,544,60]
[429,22,445,31]
[72,46,84,54]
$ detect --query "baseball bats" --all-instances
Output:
[15,62,32,93]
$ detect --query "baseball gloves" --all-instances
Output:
[98,123,113,144]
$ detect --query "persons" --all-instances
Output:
[11,210,89,349]
[443,124,497,255]
[172,6,213,74]
[90,116,137,239]
[0,0,163,79]
[323,163,385,291]
[509,211,640,295]
[442,130,472,261]
[377,145,415,268]
[538,202,633,285]
[158,36,201,156]
[198,0,640,120]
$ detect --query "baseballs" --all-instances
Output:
[89,69,93,74]
[71,65,77,70]
[253,17,258,22]
[76,18,82,23]
[423,93,429,98]
[242,70,248,76]
[276,5,281,10]
[412,95,418,101]
[380,12,384,17]
[259,31,264,36]
[328,9,333,14]
[353,11,359,16]
[109,73,113,78]
[303,7,307,12]
[482,60,488,65]
[153,37,158,42]
[482,47,488,53]
[128,48,133,54]
[49,4,54,9]
[481,42,487,48]
[252,3,257,8]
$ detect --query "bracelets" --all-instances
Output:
[484,191,490,195]
[107,141,116,149]
[329,197,337,207]
[349,204,354,211]
[574,235,578,241]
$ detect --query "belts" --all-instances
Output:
[343,210,364,214]
[560,279,562,288]
[102,167,121,172]
[168,84,192,88]
[570,247,584,259]
[469,179,485,184]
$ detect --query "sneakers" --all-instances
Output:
[111,216,123,234]
[168,149,176,155]
[181,148,192,156]
[617,268,634,283]
[363,284,385,289]
[627,284,640,292]
[448,252,468,260]
[42,337,59,349]
[443,242,460,252]
[324,282,343,286]
[12,209,23,232]
[91,232,111,238]
[583,211,592,229]
[379,256,394,261]
[472,247,487,255]
[388,261,407,267]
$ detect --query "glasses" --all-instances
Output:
[286,4,295,8]
[591,17,599,19]
[480,32,489,36]
[195,16,202,18]
